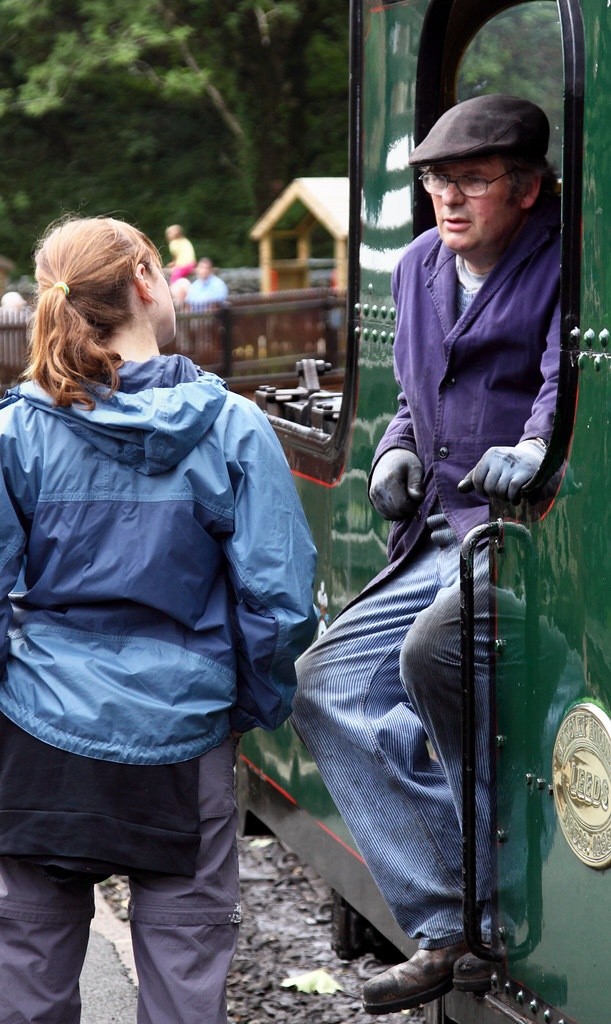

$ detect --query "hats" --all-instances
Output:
[407,92,550,168]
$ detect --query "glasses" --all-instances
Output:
[418,165,518,197]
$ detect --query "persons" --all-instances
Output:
[0,215,321,1024]
[288,93,560,1016]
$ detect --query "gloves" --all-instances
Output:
[368,448,424,521]
[457,437,549,507]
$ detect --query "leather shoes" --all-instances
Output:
[362,943,480,1016]
[452,942,504,992]
